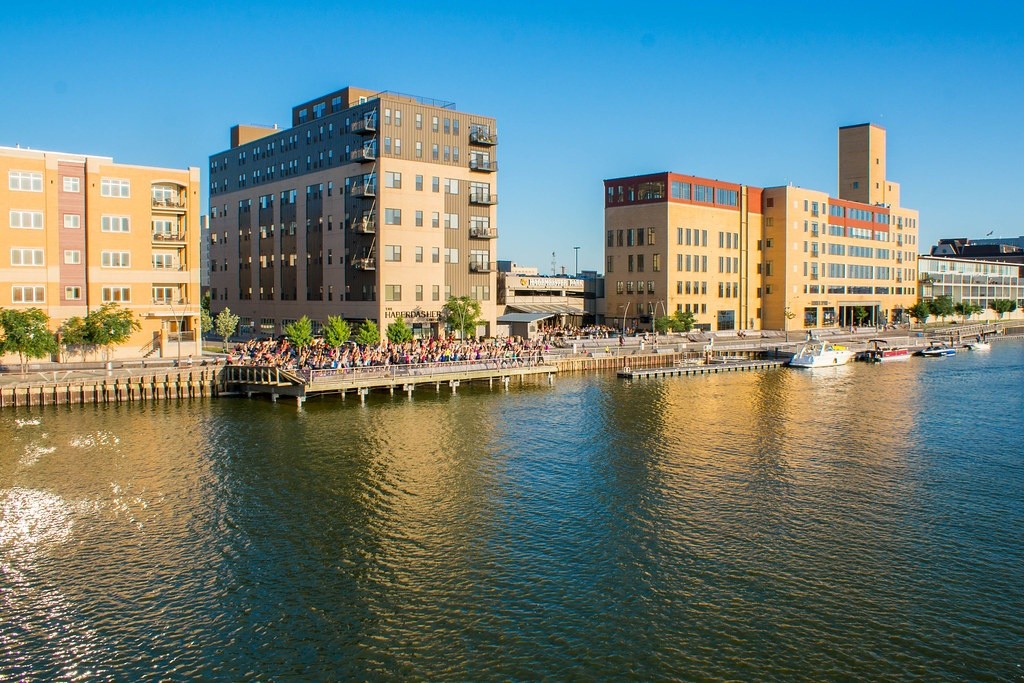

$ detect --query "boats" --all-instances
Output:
[857,339,912,365]
[789,339,856,368]
[921,341,956,357]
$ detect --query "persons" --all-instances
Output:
[737,331,746,337]
[187,322,660,381]
[805,330,812,339]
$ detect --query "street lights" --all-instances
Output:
[573,247,581,278]
